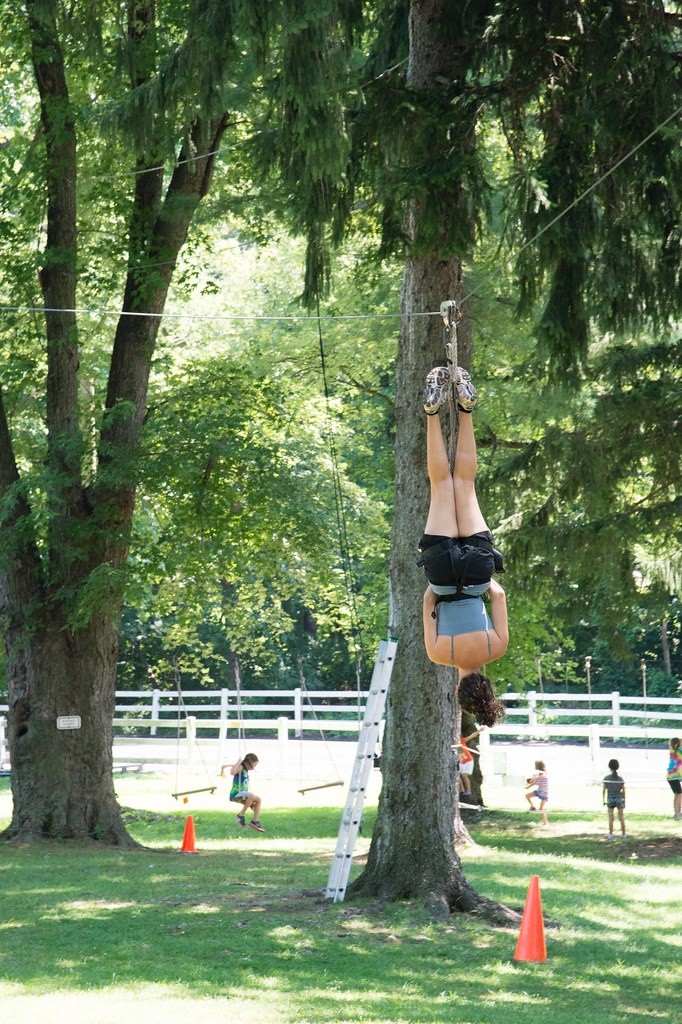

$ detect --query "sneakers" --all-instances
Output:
[422,366,452,414]
[453,366,477,411]
[249,820,265,832]
[236,814,246,827]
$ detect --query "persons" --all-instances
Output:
[422,367,509,727]
[666,737,682,820]
[219,752,265,832]
[458,727,484,795]
[524,760,552,827]
[602,759,628,839]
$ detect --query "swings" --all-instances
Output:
[229,665,258,806]
[170,681,217,800]
[297,671,345,796]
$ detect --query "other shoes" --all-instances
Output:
[606,833,613,844]
[620,835,628,841]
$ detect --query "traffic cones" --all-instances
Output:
[513,875,548,962]
[181,815,198,853]
